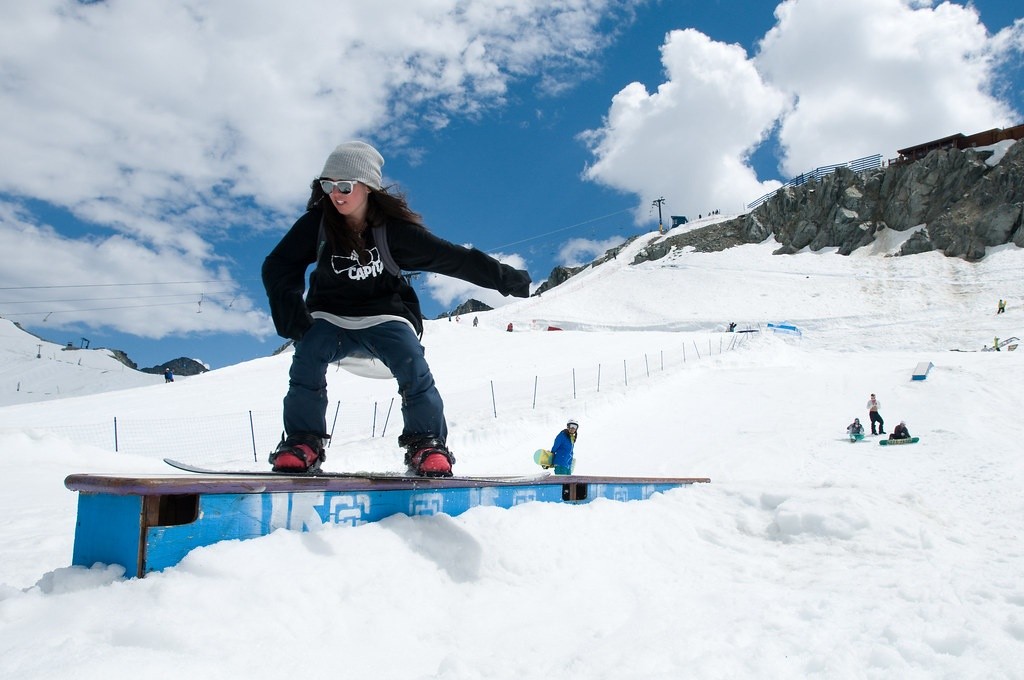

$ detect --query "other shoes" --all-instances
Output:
[872,432,878,435]
[879,431,886,434]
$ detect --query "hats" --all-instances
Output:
[320,141,384,191]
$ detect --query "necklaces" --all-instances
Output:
[354,219,366,239]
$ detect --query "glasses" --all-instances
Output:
[569,423,576,429]
[319,179,357,195]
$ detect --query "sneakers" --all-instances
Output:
[274,435,322,473]
[406,443,452,476]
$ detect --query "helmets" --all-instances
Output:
[900,421,905,424]
[567,419,579,429]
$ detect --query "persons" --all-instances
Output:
[508,323,513,332]
[889,420,911,440]
[448,313,451,321]
[847,418,865,442]
[261,141,532,478]
[455,314,460,321]
[729,322,737,332]
[165,368,174,383]
[550,418,579,475]
[866,393,886,435]
[997,300,1005,313]
[981,345,988,351]
[472,316,478,327]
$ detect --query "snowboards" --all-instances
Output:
[162,456,551,483]
